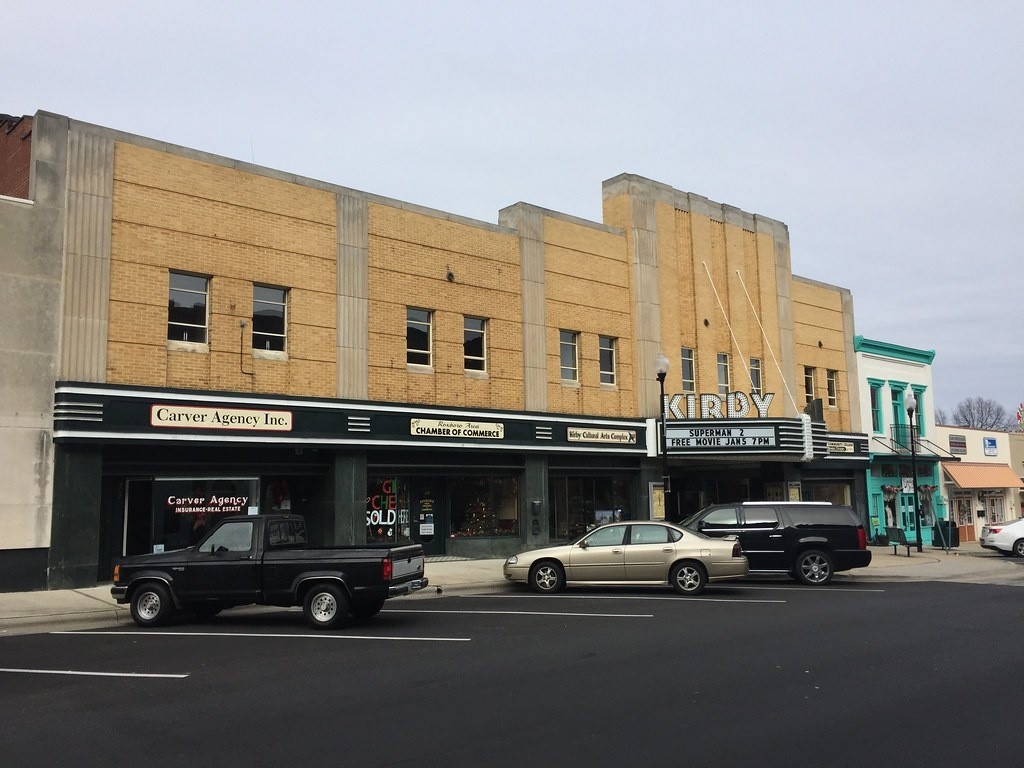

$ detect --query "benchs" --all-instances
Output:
[883,527,919,557]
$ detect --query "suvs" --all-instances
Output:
[677,500,872,585]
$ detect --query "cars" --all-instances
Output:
[977,516,1024,557]
[502,520,751,597]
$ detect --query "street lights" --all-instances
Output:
[905,391,925,552]
[652,351,671,515]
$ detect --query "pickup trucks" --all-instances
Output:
[109,513,429,629]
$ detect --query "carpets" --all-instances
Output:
[424,556,473,563]
